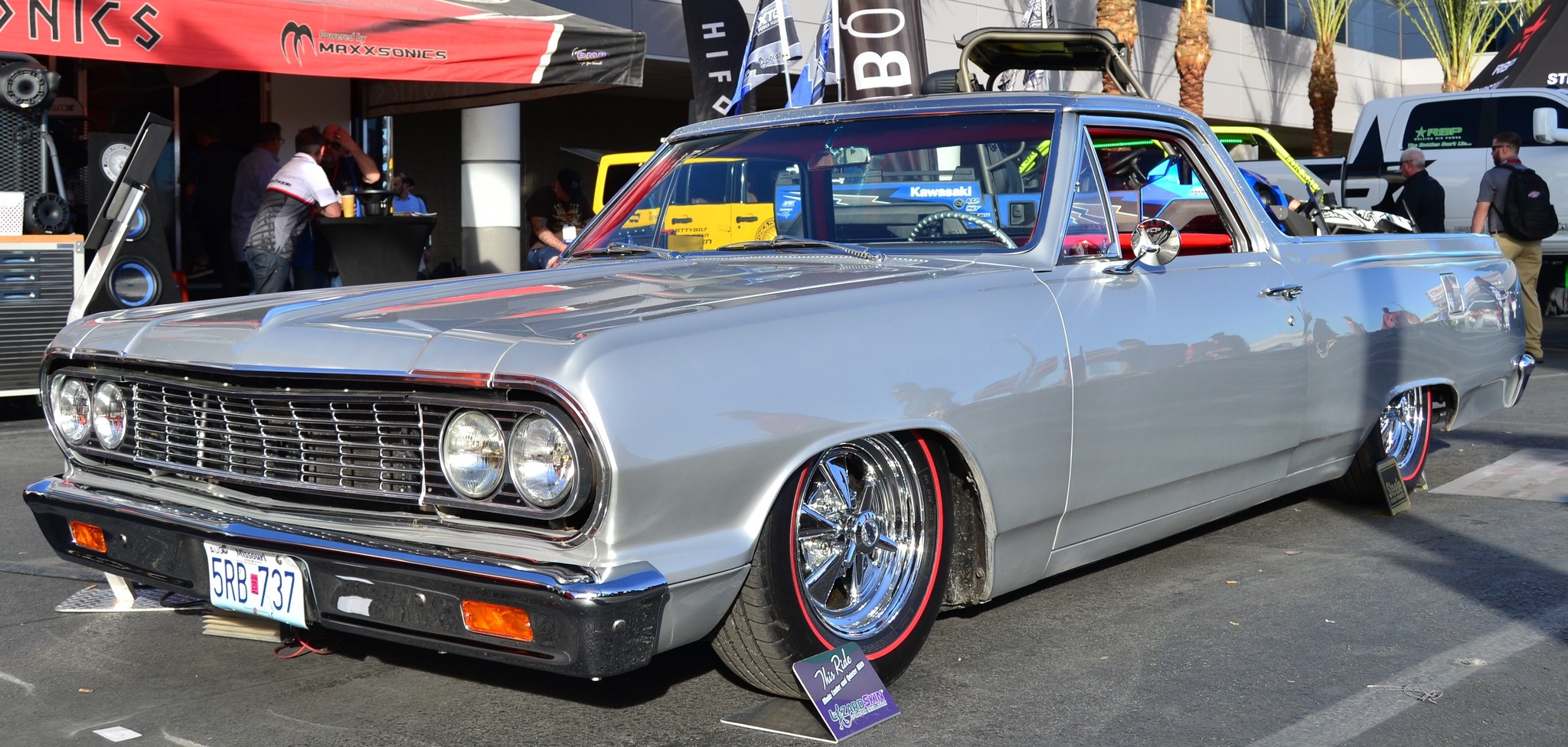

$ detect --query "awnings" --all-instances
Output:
[0,0,647,108]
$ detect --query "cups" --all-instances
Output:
[342,194,354,217]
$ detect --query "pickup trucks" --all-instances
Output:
[1232,86,1568,259]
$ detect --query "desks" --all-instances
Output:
[312,216,437,286]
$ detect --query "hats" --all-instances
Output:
[557,167,584,197]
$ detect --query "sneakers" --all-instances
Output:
[185,262,214,280]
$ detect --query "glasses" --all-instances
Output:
[1492,145,1511,151]
[271,137,285,145]
[322,137,342,150]
[1399,160,1413,166]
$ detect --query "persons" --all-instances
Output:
[524,171,591,270]
[315,120,381,290]
[391,172,428,215]
[233,121,285,300]
[1471,130,1544,362]
[1395,147,1446,235]
[1284,193,1301,212]
[243,126,343,298]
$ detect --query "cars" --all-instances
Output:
[21,88,1542,706]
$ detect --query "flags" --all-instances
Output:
[727,0,803,117]
[784,0,832,110]
[998,0,1042,92]
[1023,0,1055,91]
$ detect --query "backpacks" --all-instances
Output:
[1491,164,1559,241]
